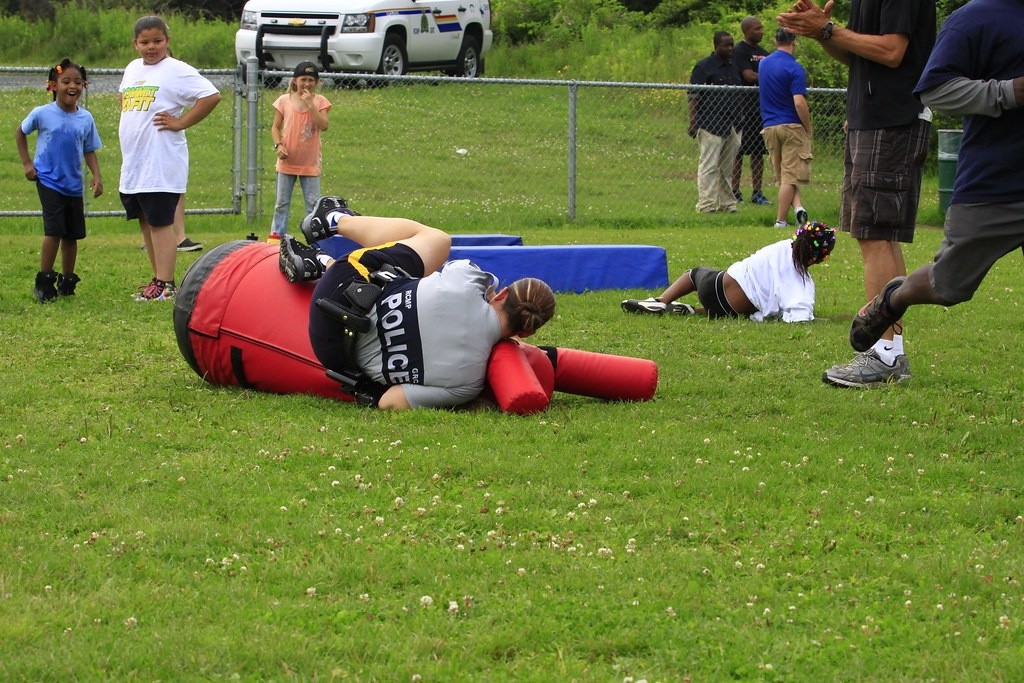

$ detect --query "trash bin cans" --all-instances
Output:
[938,130,963,214]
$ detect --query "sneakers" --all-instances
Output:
[621,298,667,318]
[794,206,807,226]
[822,348,910,389]
[850,276,908,352]
[131,278,157,298]
[668,302,695,316]
[302,196,354,245]
[774,221,790,230]
[279,234,323,282]
[733,191,744,203]
[752,191,771,205]
[135,280,176,305]
[176,237,203,252]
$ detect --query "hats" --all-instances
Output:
[293,61,318,78]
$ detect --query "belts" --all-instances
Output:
[342,278,384,370]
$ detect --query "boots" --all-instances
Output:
[35,269,60,305]
[57,273,81,297]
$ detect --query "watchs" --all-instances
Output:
[275,142,282,148]
[820,21,835,42]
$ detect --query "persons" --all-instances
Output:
[271,62,332,237]
[621,222,855,322]
[849,0,1024,353]
[732,17,772,205]
[279,196,556,411]
[17,59,103,302]
[688,31,743,214]
[775,0,934,389]
[117,16,221,303]
[759,28,812,228]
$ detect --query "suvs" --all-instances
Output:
[235,1,493,88]
